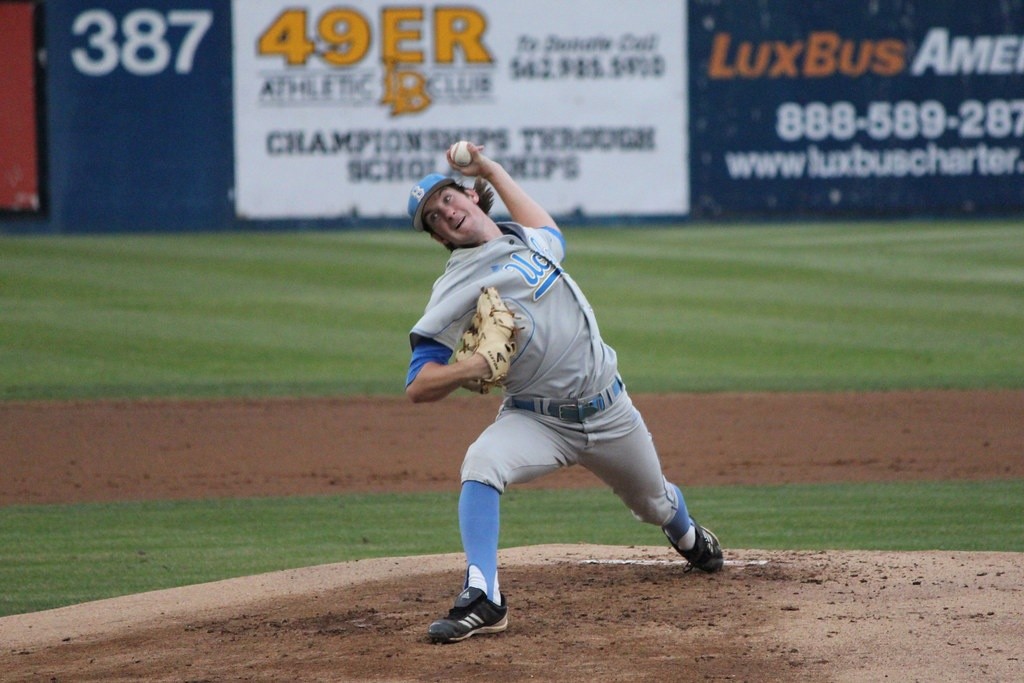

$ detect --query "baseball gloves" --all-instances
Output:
[451,286,522,396]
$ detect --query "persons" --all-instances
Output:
[406,142,724,642]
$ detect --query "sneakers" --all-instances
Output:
[428,587,508,642]
[662,516,723,574]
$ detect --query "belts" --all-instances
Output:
[512,378,621,422]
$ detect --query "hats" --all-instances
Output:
[407,173,455,232]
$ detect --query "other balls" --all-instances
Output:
[450,140,474,165]
